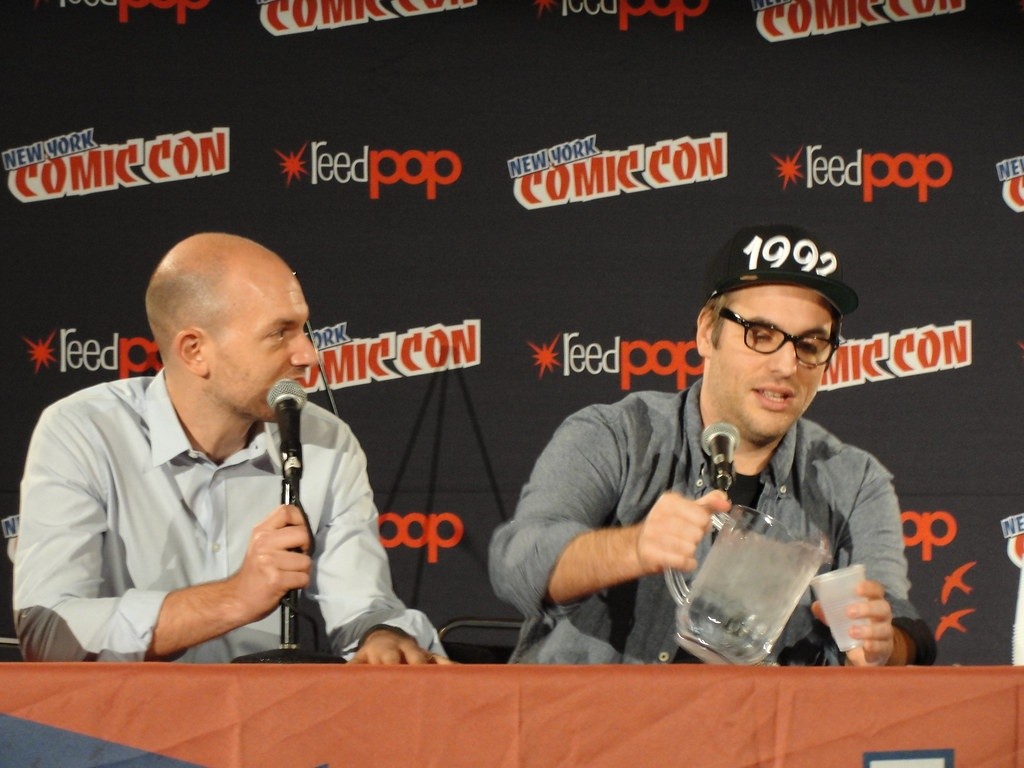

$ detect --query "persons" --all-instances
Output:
[490,225,941,669]
[10,231,450,666]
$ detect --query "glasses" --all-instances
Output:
[718,307,836,366]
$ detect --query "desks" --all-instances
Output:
[0,660,1024,768]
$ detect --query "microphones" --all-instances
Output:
[701,422,740,493]
[266,378,306,482]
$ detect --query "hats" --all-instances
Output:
[707,225,859,339]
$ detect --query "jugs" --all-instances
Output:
[665,505,832,664]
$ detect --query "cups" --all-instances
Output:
[809,564,872,652]
